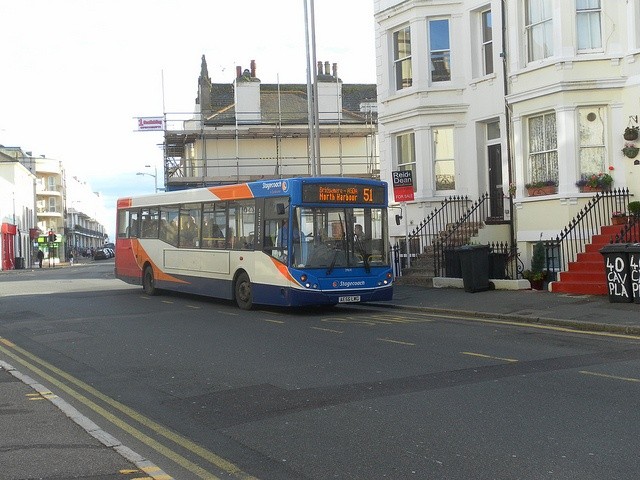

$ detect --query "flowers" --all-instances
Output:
[606,165,615,177]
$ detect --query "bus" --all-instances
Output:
[113,176,394,313]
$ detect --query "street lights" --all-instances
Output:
[393,171,414,202]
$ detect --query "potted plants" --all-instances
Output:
[526,180,556,196]
[626,201,640,226]
[576,173,613,193]
[611,211,627,225]
[534,272,544,290]
[624,126,639,140]
[622,142,639,158]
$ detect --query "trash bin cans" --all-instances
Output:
[627,243,640,304]
[489,253,506,279]
[22,258,24,269]
[15,257,22,269]
[457,244,490,292]
[598,243,633,303]
[444,248,461,278]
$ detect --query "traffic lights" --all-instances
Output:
[49,231,53,241]
[54,234,57,242]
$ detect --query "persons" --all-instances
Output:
[355,224,365,241]
[37,250,44,268]
[279,218,306,250]
[69,251,74,266]
